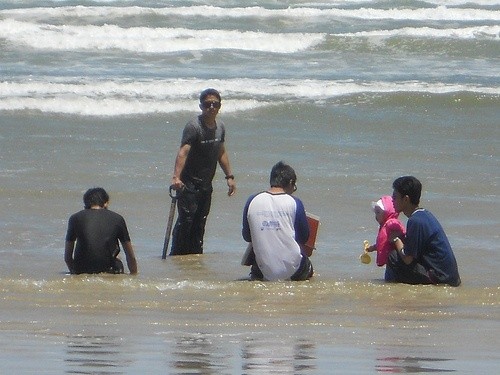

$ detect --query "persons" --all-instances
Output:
[389,175,461,288]
[170,87,239,256]
[63,187,137,274]
[241,161,315,281]
[365,195,407,281]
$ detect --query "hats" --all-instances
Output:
[371,199,384,211]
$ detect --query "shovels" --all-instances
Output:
[361,240,371,264]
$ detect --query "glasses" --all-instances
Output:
[294,184,297,191]
[202,101,221,109]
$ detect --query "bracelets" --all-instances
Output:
[392,236,399,244]
[225,175,234,180]
